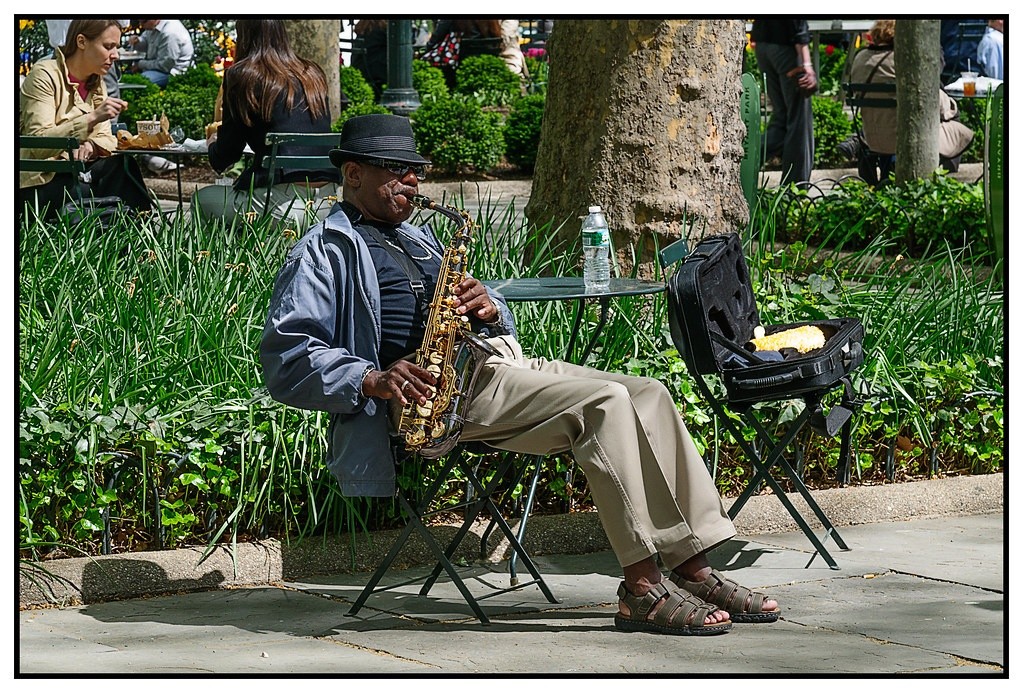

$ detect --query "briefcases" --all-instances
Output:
[669,233,867,405]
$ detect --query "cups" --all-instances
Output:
[960,72,979,96]
[135,120,161,135]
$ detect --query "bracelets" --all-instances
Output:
[803,63,812,67]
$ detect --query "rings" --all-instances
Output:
[400,380,410,391]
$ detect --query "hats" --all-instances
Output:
[329,115,431,167]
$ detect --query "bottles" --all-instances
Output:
[581,205,611,289]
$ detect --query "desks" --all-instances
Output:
[948,92,994,185]
[113,148,220,225]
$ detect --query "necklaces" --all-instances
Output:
[386,227,433,260]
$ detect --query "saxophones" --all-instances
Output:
[381,191,506,460]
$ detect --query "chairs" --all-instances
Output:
[341,441,562,628]
[840,82,898,178]
[658,238,864,572]
[19,136,89,226]
[262,132,342,221]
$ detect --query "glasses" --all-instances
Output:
[347,158,426,181]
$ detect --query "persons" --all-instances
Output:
[422,17,1003,199]
[20,20,195,233]
[258,113,782,637]
[191,20,338,236]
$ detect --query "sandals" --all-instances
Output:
[670,567,782,623]
[615,576,732,633]
